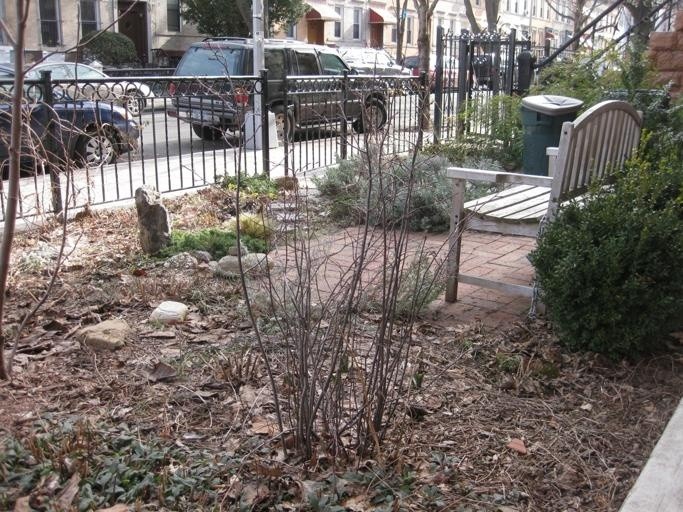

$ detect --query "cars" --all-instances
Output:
[0,60,156,117]
[1,86,140,169]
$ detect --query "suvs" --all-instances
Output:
[336,47,413,95]
[167,34,391,145]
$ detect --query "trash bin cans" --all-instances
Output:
[520,95,584,177]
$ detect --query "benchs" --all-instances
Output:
[447,101,643,315]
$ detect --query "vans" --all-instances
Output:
[466,52,517,90]
[399,54,477,93]
